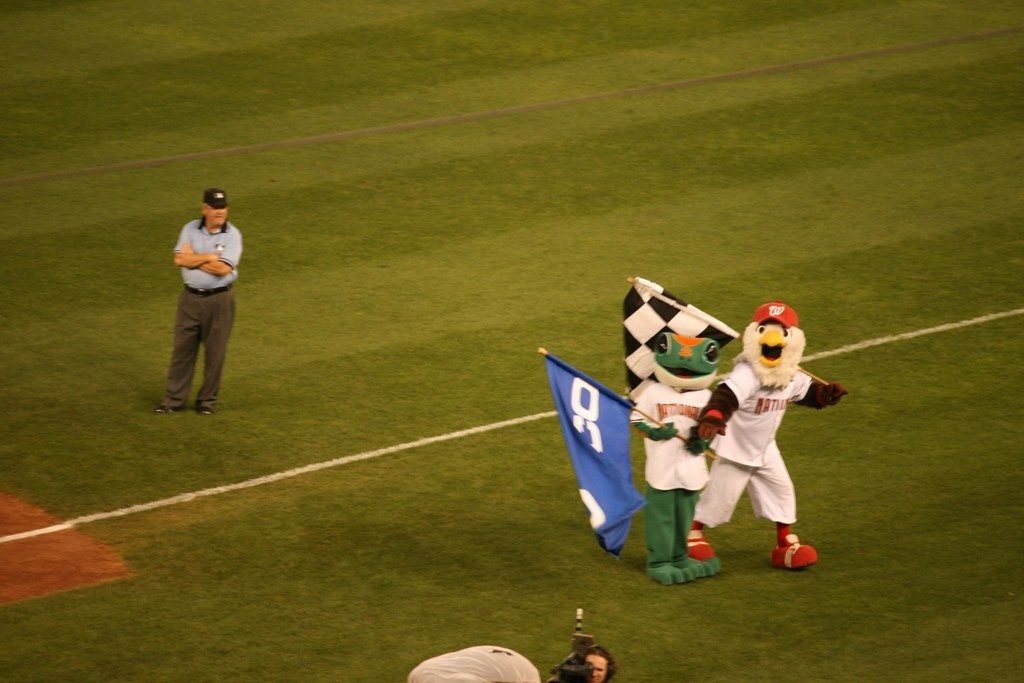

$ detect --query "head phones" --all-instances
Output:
[584,646,616,679]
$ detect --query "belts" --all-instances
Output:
[183,284,232,296]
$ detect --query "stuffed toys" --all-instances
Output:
[688,300,848,570]
[629,333,720,584]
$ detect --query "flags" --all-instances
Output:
[621,277,740,405]
[545,354,649,560]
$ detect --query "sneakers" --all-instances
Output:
[197,407,214,415]
[155,405,186,413]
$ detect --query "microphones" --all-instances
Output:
[551,652,576,675]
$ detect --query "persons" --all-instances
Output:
[585,645,619,683]
[155,189,244,414]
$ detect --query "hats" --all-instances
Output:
[203,187,228,208]
